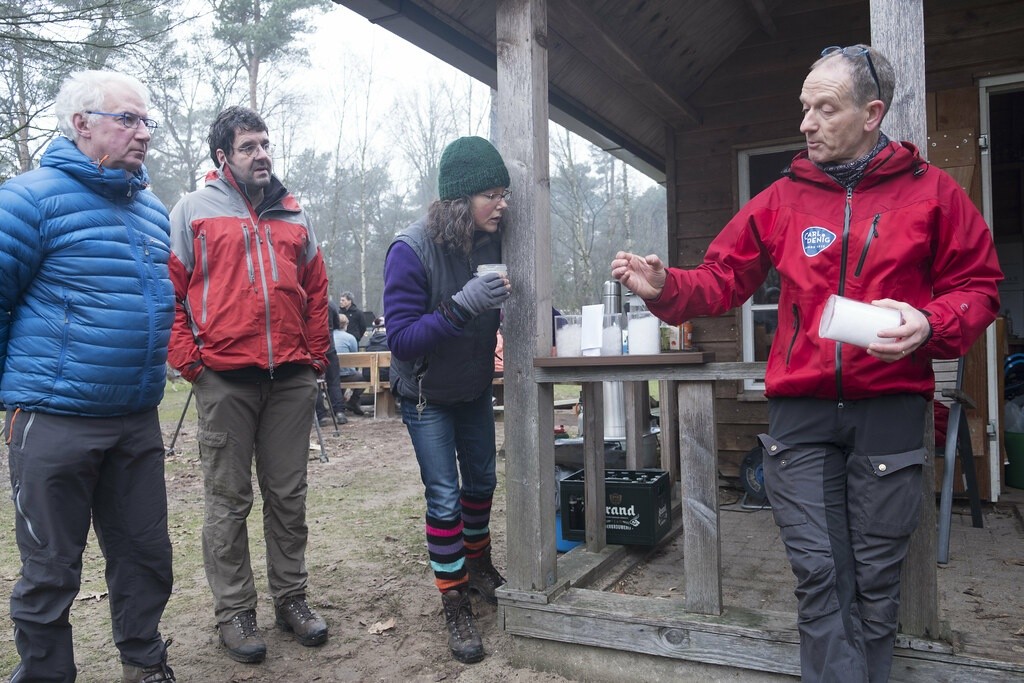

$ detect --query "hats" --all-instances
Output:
[372,316,384,330]
[438,136,510,202]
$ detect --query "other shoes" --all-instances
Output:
[336,412,347,424]
[344,401,364,416]
[319,417,330,427]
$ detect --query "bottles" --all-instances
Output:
[606,472,662,483]
[569,476,585,530]
[576,391,584,438]
[554,425,578,440]
[678,320,692,349]
[602,280,621,314]
[624,290,650,316]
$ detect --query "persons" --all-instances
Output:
[168,106,332,665]
[0,70,176,683]
[552,307,568,356]
[333,314,367,415]
[492,327,503,405]
[611,44,1006,683]
[338,291,366,341]
[315,302,348,426]
[362,316,401,409]
[382,136,511,663]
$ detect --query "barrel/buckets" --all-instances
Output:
[1004,430,1024,490]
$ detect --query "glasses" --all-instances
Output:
[478,189,513,203]
[232,143,276,158]
[84,111,158,134]
[821,46,881,100]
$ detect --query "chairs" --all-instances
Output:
[919,349,983,560]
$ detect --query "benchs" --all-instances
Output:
[336,352,400,418]
[492,377,505,415]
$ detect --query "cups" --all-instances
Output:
[602,314,623,357]
[627,311,661,355]
[818,294,901,352]
[478,264,507,309]
[660,327,670,352]
[554,315,582,357]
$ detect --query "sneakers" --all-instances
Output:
[441,587,485,664]
[121,639,177,683]
[275,594,328,646]
[466,544,508,605]
[216,609,267,662]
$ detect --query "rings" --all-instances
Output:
[902,351,905,356]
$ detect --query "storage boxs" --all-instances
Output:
[560,469,672,546]
